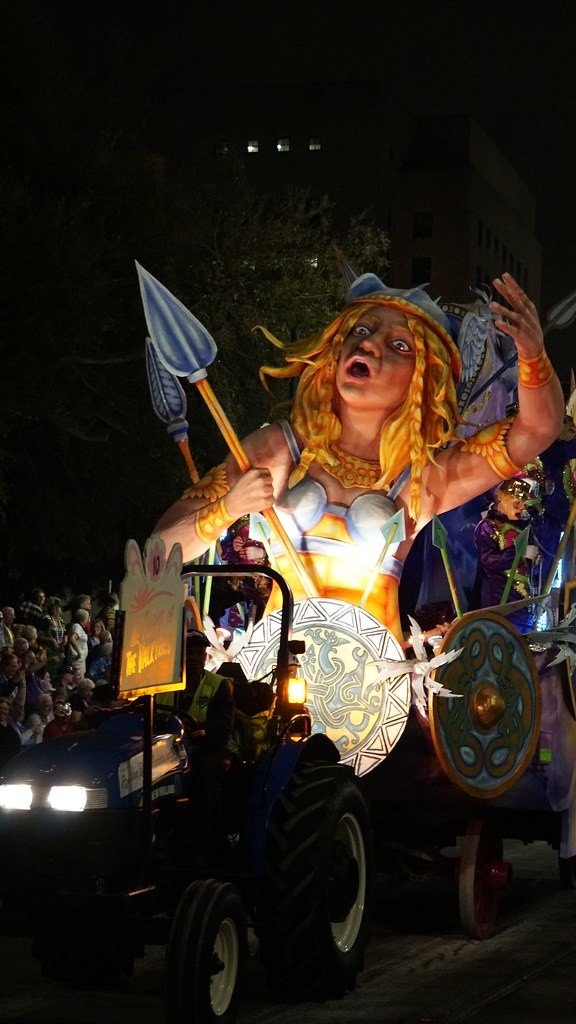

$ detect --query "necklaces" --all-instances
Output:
[319,443,402,488]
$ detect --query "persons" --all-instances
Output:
[0,570,269,766]
[149,275,566,647]
[206,517,270,626]
[139,629,235,859]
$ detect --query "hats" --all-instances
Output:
[41,680,57,691]
[0,611,3,618]
[187,629,210,647]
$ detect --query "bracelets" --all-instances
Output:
[518,348,553,387]
[196,498,235,545]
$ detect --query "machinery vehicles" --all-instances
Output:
[0,564,384,1022]
